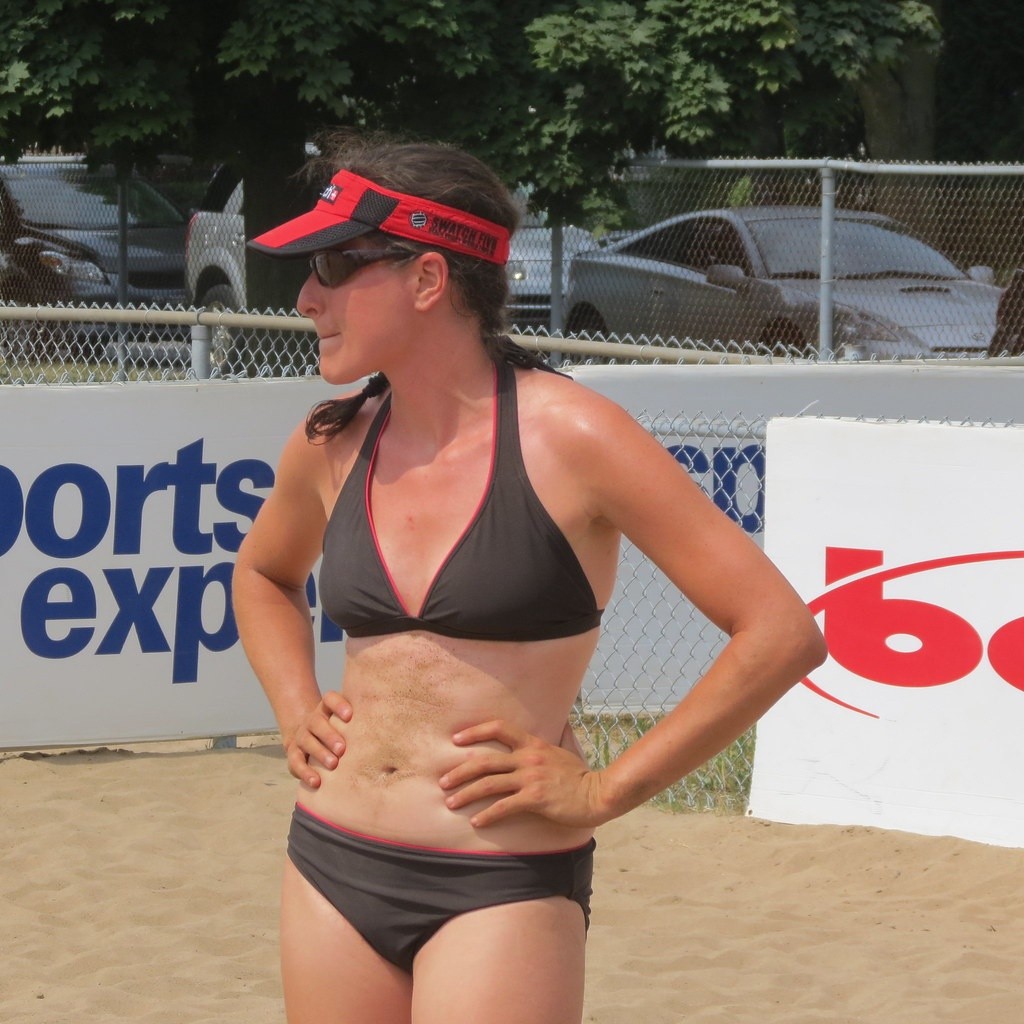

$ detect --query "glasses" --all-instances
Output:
[308,246,415,288]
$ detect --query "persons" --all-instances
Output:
[232,140,829,1024]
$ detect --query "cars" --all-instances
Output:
[0,150,601,379]
[564,206,1007,361]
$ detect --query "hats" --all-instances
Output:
[246,169,511,265]
